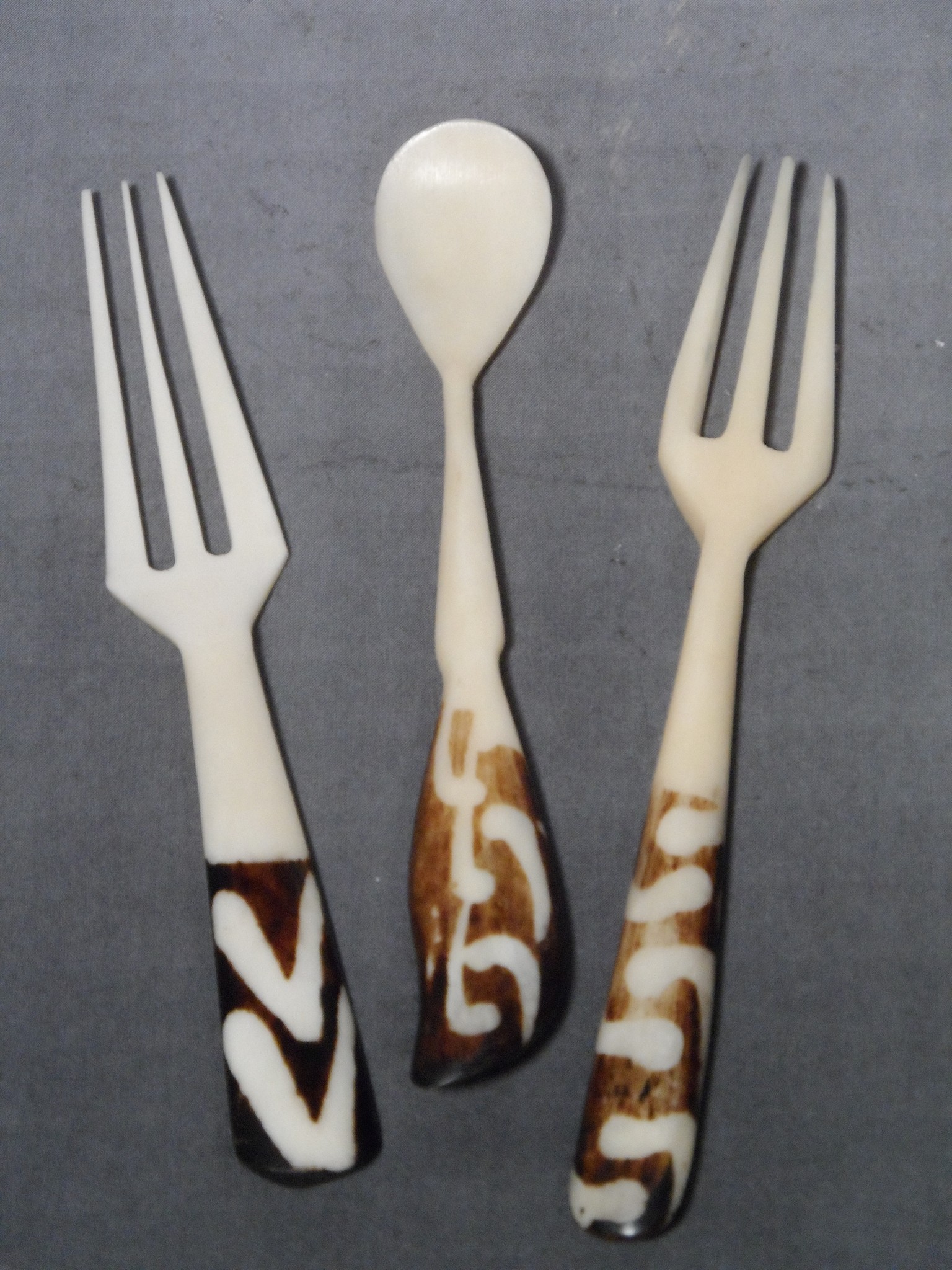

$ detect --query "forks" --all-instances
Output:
[567,150,837,1238]
[81,172,384,1189]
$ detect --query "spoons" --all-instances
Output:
[374,119,578,1085]
